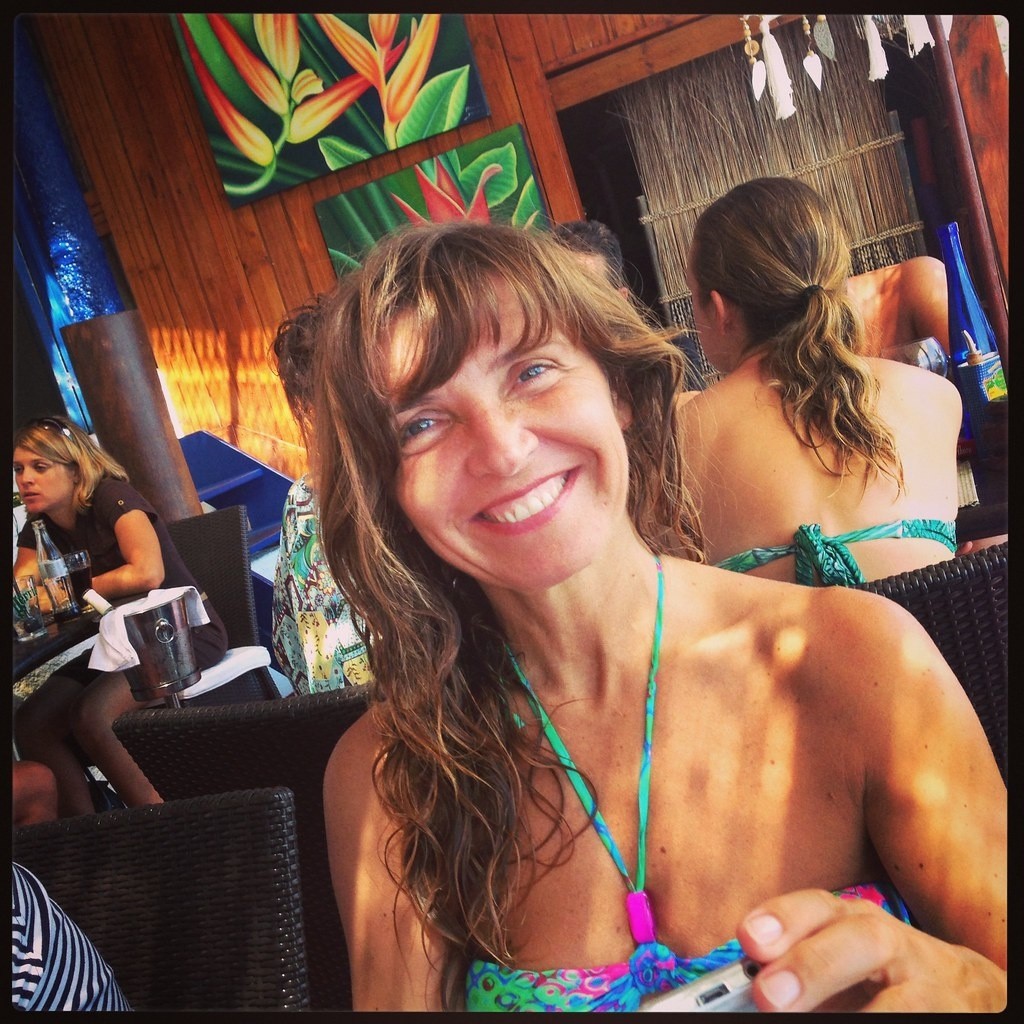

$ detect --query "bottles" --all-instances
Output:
[82,588,115,617]
[31,520,80,616]
[932,221,1008,458]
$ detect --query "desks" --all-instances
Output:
[12,592,146,763]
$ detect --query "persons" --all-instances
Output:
[834,255,949,371]
[267,222,1008,1012]
[272,217,700,688]
[11,862,136,1011]
[672,177,964,587]
[12,414,230,827]
[12,762,57,826]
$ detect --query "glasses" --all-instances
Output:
[24,416,78,445]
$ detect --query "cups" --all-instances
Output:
[12,575,48,642]
[877,337,948,382]
[64,550,96,613]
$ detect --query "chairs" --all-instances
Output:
[111,682,373,1015]
[12,785,313,1015]
[166,505,285,702]
[833,541,1008,779]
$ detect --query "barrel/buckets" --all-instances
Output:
[103,593,202,702]
